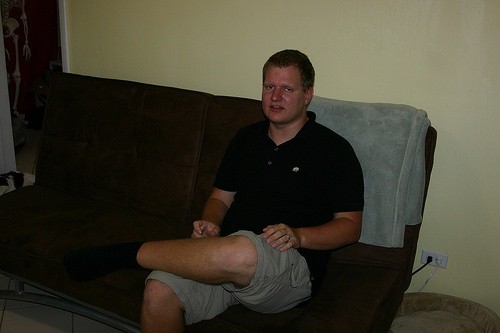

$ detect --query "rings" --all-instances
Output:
[285,234,290,241]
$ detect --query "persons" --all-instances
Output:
[63,50,365,332]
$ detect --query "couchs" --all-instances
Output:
[0,72,437,333]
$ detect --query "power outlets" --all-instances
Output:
[421,249,448,269]
